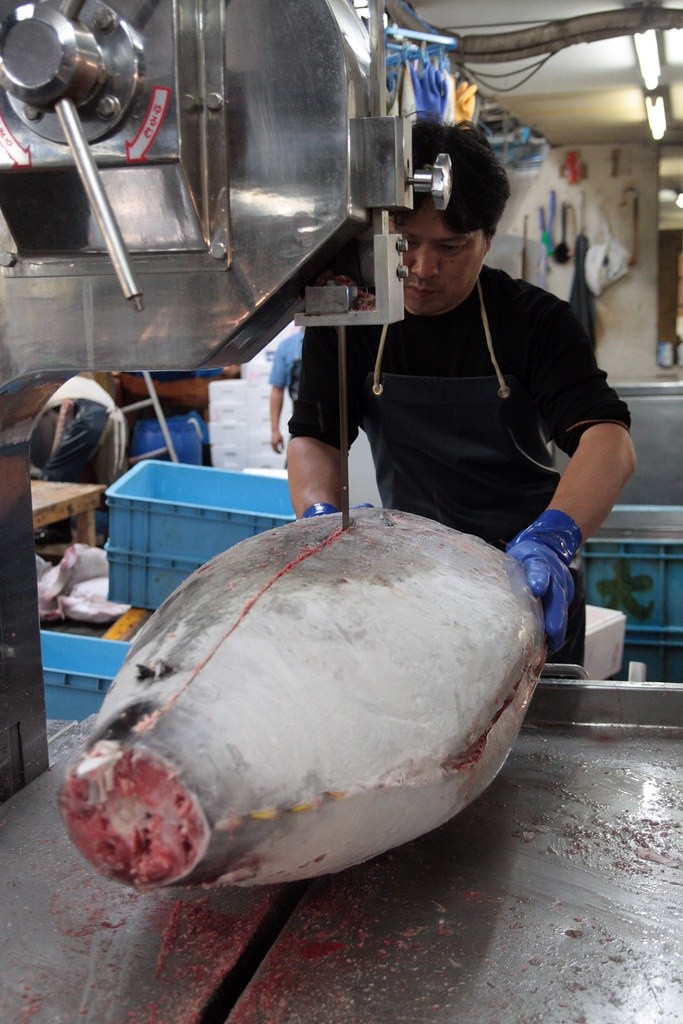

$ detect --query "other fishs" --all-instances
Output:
[57,506,548,892]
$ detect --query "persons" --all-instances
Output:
[266,115,638,661]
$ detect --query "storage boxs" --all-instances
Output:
[580,537,683,683]
[40,629,134,721]
[104,460,296,611]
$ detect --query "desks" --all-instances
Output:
[31,479,107,547]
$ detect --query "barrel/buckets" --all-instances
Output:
[131,418,203,465]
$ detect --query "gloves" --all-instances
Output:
[504,510,581,655]
[304,502,375,518]
